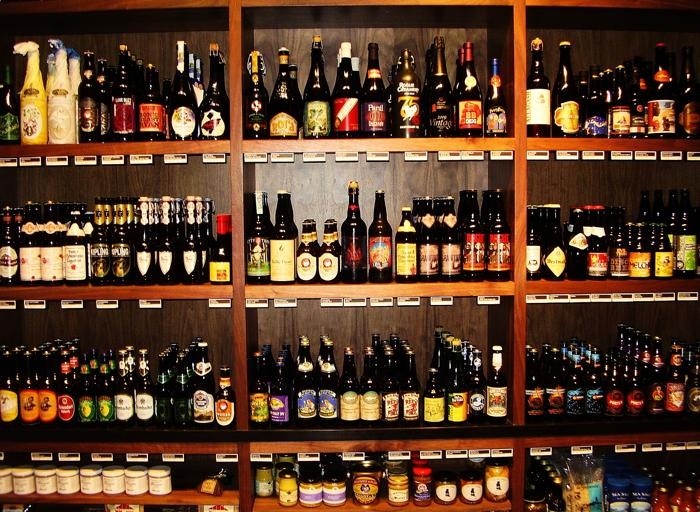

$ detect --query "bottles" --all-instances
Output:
[524,452,566,512]
[255,453,299,507]
[648,459,700,512]
[0,320,700,430]
[0,35,699,139]
[0,185,700,285]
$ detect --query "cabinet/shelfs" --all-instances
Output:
[0,0,699,512]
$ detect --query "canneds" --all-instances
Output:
[0,462,173,496]
[255,454,511,508]
[603,461,653,512]
[523,490,549,512]
